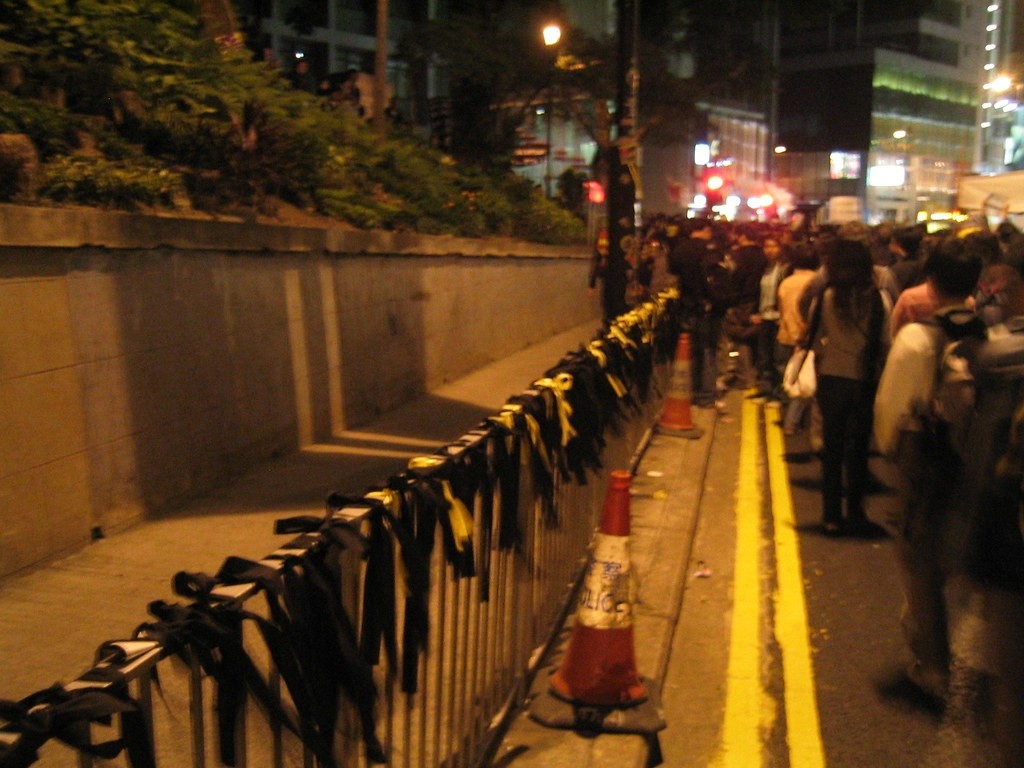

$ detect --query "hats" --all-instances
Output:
[893,225,924,252]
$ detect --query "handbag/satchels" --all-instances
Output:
[780,286,817,399]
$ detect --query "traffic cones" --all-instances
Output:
[652,331,704,441]
[530,470,668,733]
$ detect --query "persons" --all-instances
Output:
[589,209,635,329]
[630,206,1024,728]
